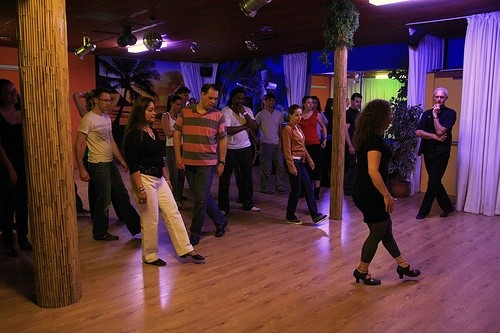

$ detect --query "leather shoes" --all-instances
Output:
[94,232,119,241]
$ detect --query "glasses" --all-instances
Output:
[98,97,112,102]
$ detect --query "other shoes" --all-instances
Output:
[0,222,17,232]
[0,245,18,256]
[300,190,304,198]
[259,189,274,194]
[416,214,426,219]
[220,211,225,215]
[175,200,184,210]
[440,208,456,218]
[314,193,320,200]
[275,187,288,193]
[251,207,261,211]
[19,240,33,250]
[78,208,91,215]
[235,196,257,204]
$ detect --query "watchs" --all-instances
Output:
[219,160,226,164]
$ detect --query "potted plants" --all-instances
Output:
[385,96,423,197]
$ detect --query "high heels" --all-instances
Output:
[189,233,199,246]
[143,258,166,266]
[396,264,421,279]
[215,219,228,237]
[352,269,381,286]
[180,250,205,260]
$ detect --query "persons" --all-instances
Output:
[282,103,327,224]
[415,87,457,219]
[173,83,228,245]
[344,92,362,194]
[174,85,191,105]
[74,183,90,216]
[161,94,188,208]
[123,97,204,267]
[353,98,420,286]
[324,97,333,134]
[251,92,290,194]
[74,89,141,240]
[346,98,349,109]
[72,88,130,222]
[298,95,327,199]
[312,95,328,138]
[218,86,261,216]
[0,78,33,256]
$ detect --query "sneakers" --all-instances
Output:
[286,219,304,225]
[313,214,328,225]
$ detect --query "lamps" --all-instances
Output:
[190,42,199,53]
[117,26,138,48]
[143,19,164,51]
[75,36,97,58]
[240,0,275,50]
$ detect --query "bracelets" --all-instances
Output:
[433,114,438,118]
[137,185,145,191]
